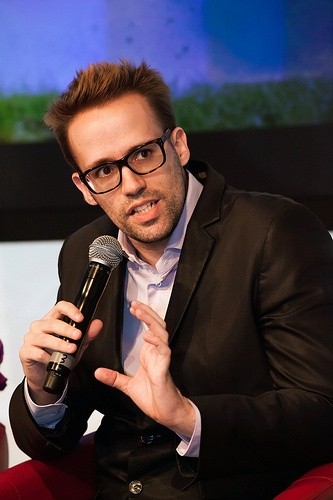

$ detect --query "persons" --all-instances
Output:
[8,61,333,500]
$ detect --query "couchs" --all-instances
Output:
[0,459,333,500]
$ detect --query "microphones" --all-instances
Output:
[42,235,123,394]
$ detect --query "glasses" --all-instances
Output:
[77,128,170,194]
